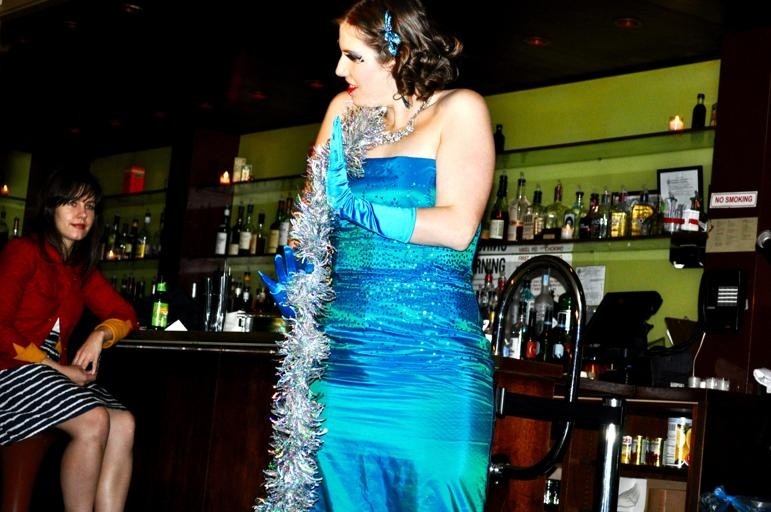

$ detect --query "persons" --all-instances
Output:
[257,3,495,511]
[0,168,136,512]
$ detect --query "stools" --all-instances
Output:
[2,426,61,512]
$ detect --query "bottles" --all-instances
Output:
[473,172,677,244]
[691,197,706,231]
[691,94,707,129]
[100,198,291,267]
[474,270,585,365]
[105,268,293,331]
[617,416,693,469]
[493,123,505,151]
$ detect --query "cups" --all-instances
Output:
[681,209,702,232]
[667,115,686,132]
[219,171,230,184]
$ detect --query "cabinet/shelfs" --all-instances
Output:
[222,125,730,268]
[609,398,707,512]
[90,185,167,269]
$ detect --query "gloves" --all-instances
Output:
[323,113,417,244]
[258,243,310,323]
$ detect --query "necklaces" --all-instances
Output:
[370,95,428,145]
